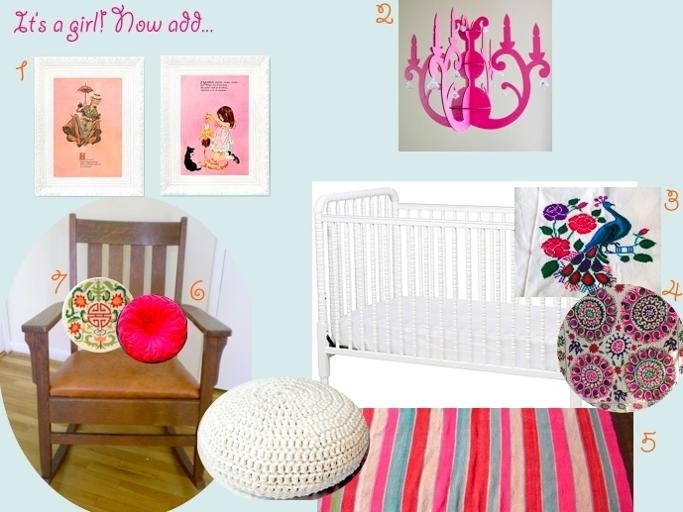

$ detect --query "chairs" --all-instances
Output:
[23,214,232,495]
